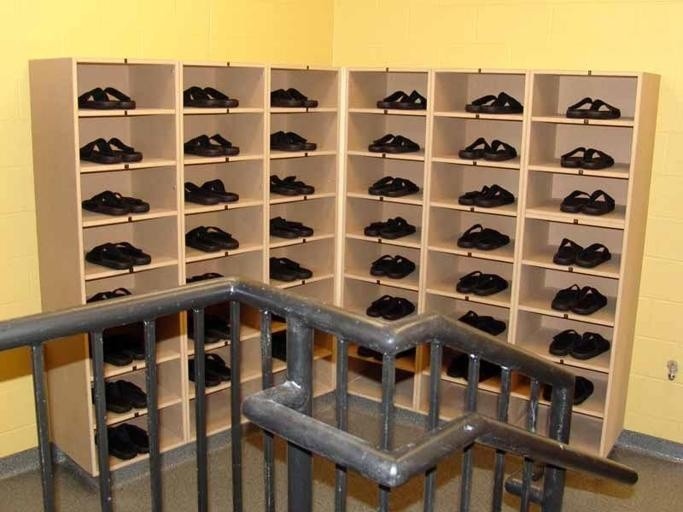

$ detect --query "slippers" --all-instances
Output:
[357,89,621,406]
[78,86,318,460]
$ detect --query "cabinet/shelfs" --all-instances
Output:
[26,58,341,480]
[340,64,662,462]
